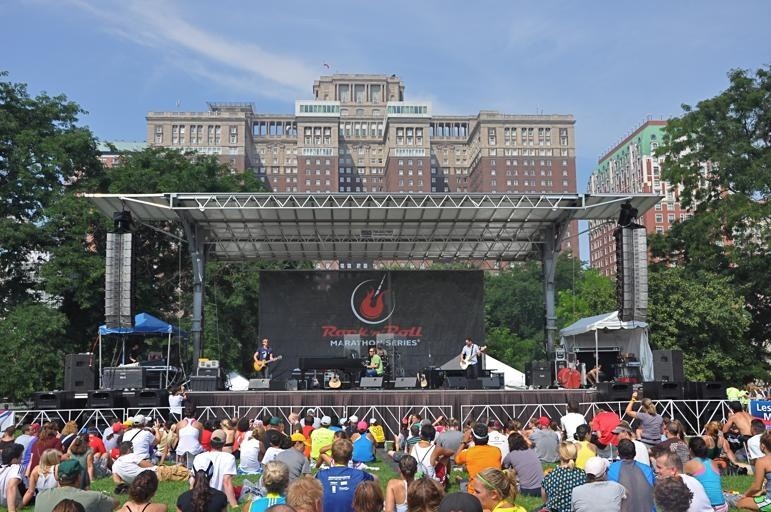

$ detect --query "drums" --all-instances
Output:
[558,368,581,389]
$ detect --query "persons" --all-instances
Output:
[365,346,384,378]
[586,350,606,389]
[127,343,139,364]
[254,338,275,378]
[461,337,481,381]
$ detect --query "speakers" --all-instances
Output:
[471,377,501,389]
[441,377,469,389]
[88,391,121,426]
[249,378,271,389]
[33,390,66,425]
[642,381,689,433]
[615,227,649,323]
[654,348,685,381]
[104,232,135,330]
[525,361,550,387]
[688,381,726,430]
[135,388,169,423]
[64,353,95,393]
[599,382,634,421]
[359,377,383,388]
[395,377,418,388]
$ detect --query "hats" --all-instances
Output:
[210,428,229,445]
[610,425,632,434]
[584,457,610,479]
[112,423,127,433]
[269,416,285,425]
[306,408,332,426]
[289,433,312,447]
[339,415,377,431]
[131,416,153,426]
[191,456,213,478]
[57,458,86,481]
[536,416,550,426]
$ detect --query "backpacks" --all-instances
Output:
[413,443,435,480]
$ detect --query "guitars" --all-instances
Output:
[329,369,341,388]
[253,356,283,371]
[459,346,487,371]
